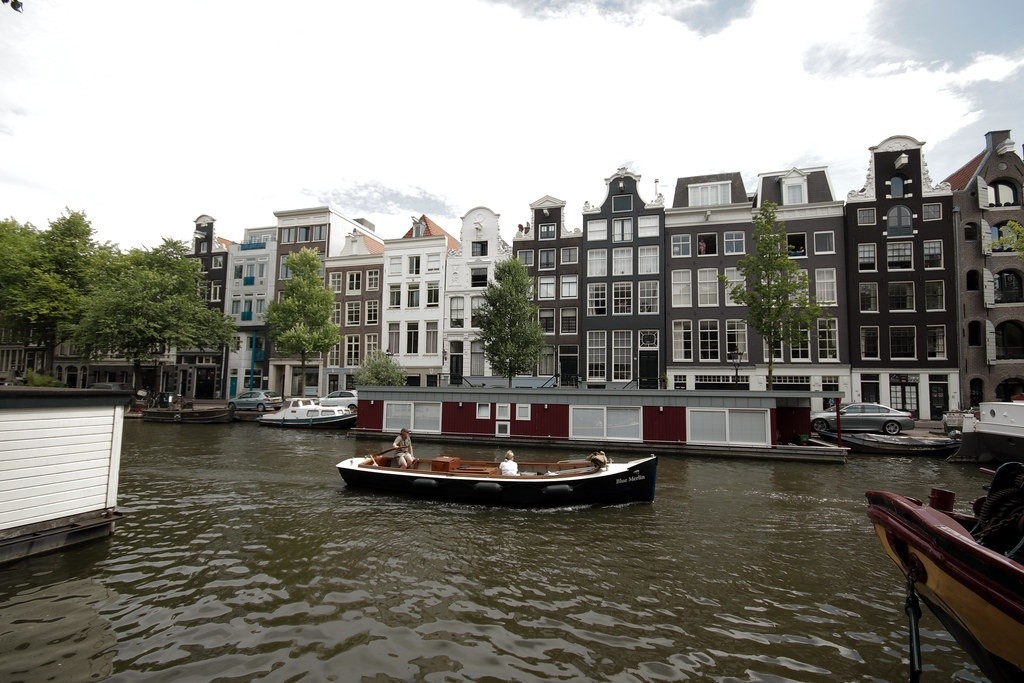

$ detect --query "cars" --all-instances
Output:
[810,402,914,436]
[315,390,358,412]
[227,390,283,412]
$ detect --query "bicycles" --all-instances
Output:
[143,386,161,409]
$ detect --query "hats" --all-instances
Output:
[401,428,411,434]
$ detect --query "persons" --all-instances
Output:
[698,239,706,254]
[499,450,519,475]
[393,428,415,468]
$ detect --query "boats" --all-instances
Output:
[0,384,134,566]
[255,398,357,429]
[865,462,1024,683]
[334,448,657,509]
